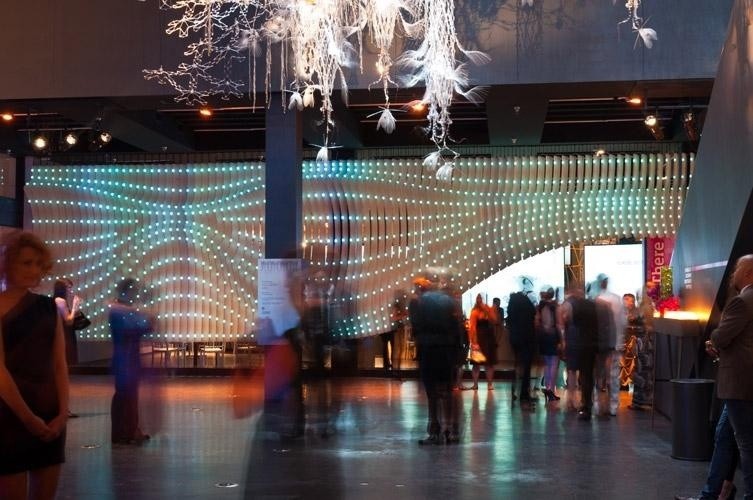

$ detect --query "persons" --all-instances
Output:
[0,229,81,500]
[674,254,753,500]
[232,242,363,441]
[108,278,157,444]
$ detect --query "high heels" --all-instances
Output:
[541,388,561,402]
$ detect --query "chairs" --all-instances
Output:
[149,340,262,368]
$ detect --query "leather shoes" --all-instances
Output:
[578,408,592,421]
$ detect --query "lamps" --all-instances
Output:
[645,115,666,142]
[629,80,644,108]
[141,0,489,181]
[681,111,701,143]
[18,127,113,150]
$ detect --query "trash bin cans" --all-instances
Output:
[670,378,715,462]
